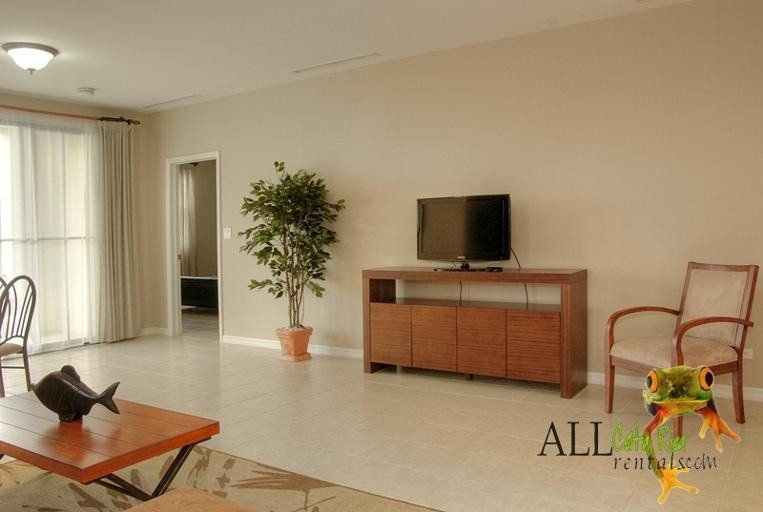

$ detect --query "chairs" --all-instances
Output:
[0,275,37,397]
[603,259,760,438]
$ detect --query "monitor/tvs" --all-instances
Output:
[417,194,511,272]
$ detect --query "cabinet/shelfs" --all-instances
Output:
[361,266,588,400]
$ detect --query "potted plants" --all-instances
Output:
[239,156,346,364]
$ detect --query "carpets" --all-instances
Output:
[0,447,436,512]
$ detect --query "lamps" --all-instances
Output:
[1,41,61,77]
